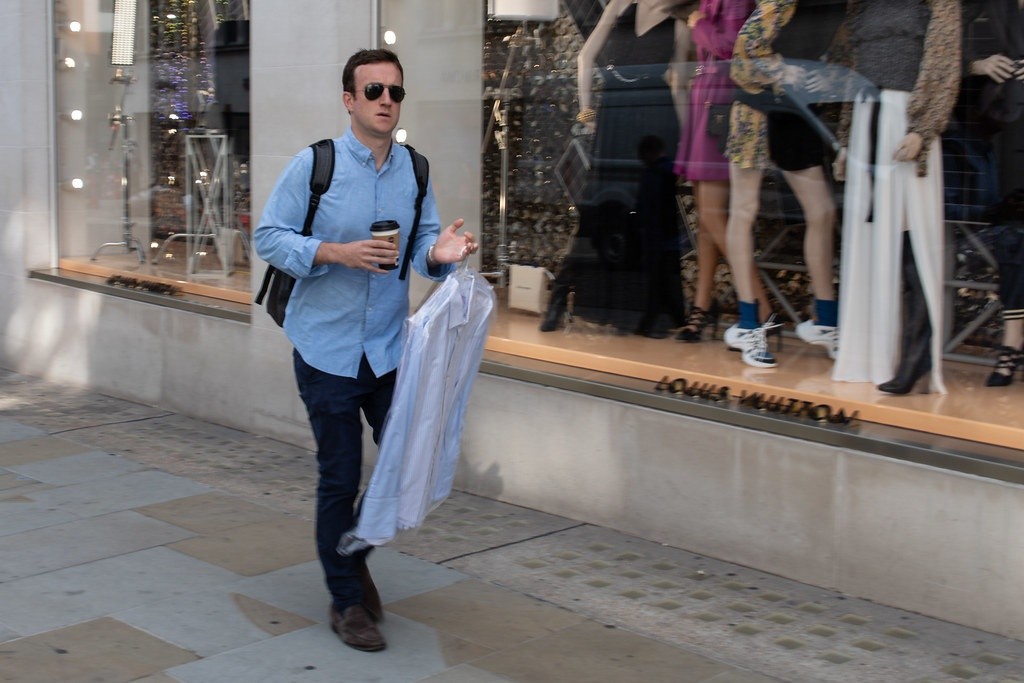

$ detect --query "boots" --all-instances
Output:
[538,251,574,332]
[877,229,933,395]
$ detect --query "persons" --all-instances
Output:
[252,48,481,651]
[535,0,1024,399]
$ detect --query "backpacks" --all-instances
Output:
[255,138,430,329]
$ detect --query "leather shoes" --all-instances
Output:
[358,560,384,622]
[330,600,386,650]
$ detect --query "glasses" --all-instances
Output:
[354,82,408,104]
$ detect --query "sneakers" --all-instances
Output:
[724,324,779,368]
[795,320,839,359]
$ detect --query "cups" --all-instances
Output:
[370,221,400,271]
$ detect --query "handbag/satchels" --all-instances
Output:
[705,102,734,135]
[765,93,840,171]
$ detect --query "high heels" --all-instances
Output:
[985,344,1024,387]
[727,311,784,353]
[676,296,721,341]
